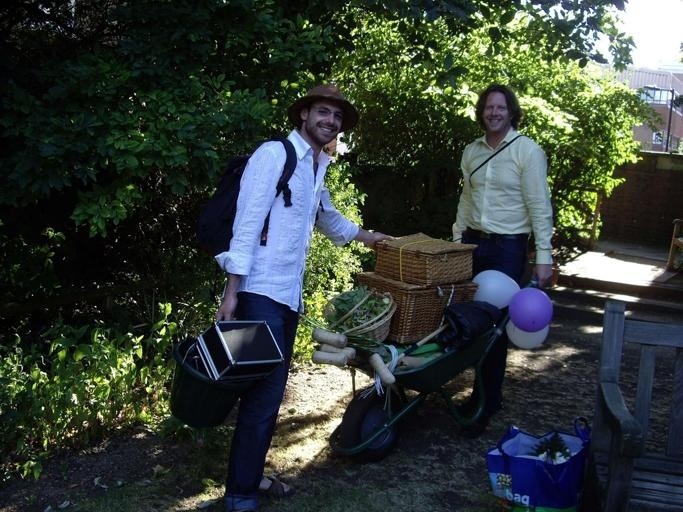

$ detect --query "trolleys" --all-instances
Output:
[328,276,537,465]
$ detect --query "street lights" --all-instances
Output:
[645,85,674,152]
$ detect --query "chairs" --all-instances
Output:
[584,300,683,512]
[666,218,683,271]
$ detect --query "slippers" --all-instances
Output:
[257,476,296,498]
[224,496,256,512]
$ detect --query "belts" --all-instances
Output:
[464,227,529,240]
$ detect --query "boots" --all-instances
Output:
[450,346,508,438]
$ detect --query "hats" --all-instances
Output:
[288,86,358,131]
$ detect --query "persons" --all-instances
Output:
[453,83,555,430]
[213,85,393,512]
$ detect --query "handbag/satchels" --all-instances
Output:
[485,417,591,511]
[444,301,499,345]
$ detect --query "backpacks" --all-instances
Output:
[198,138,297,255]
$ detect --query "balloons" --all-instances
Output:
[473,270,553,351]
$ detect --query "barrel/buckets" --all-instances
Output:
[169,340,240,428]
